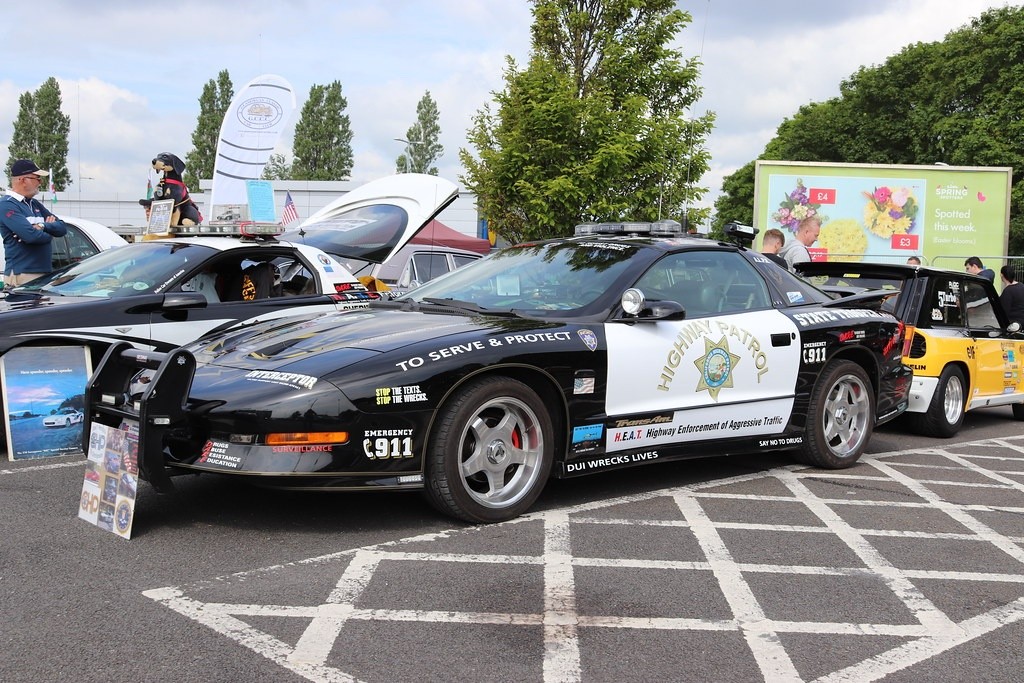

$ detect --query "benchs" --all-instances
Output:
[714,283,762,313]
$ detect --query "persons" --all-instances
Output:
[999,265,1024,333]
[778,217,824,285]
[0,159,67,300]
[906,256,922,271]
[758,229,788,276]
[964,256,995,301]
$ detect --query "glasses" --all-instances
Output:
[19,177,42,184]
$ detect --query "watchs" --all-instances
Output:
[38,223,46,230]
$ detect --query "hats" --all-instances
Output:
[11,160,50,176]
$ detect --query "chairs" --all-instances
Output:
[240,264,283,300]
[661,278,714,317]
[934,301,963,325]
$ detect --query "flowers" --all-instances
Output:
[772,179,821,235]
[865,187,919,240]
[818,219,869,263]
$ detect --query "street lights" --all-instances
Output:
[393,137,425,172]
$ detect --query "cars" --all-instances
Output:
[374,244,488,291]
[84,218,916,525]
[43,408,86,427]
[0,215,135,289]
[785,260,1024,439]
[0,172,464,439]
